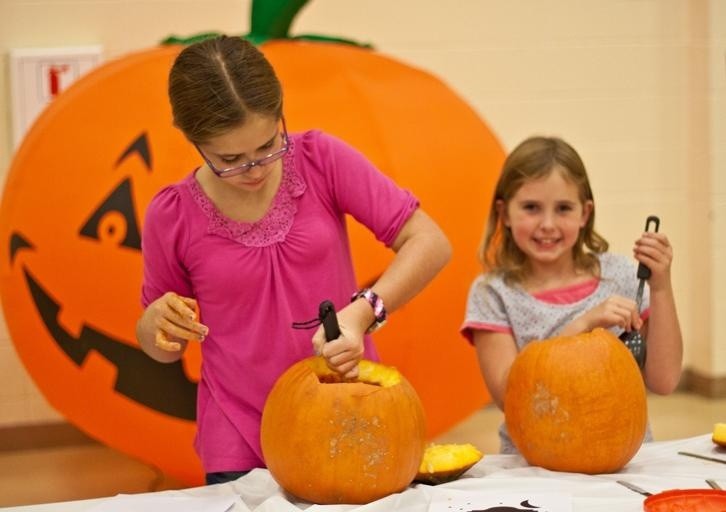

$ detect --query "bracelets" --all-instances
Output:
[350,287,389,336]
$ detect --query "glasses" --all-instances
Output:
[195,114,291,179]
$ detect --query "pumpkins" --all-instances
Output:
[503,326,646,474]
[0,0,510,485]
[260,357,485,504]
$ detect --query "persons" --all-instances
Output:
[134,35,456,491]
[458,135,688,456]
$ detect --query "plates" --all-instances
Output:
[643,490,726,512]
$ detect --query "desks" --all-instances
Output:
[0,434,726,512]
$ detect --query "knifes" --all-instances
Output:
[616,479,651,501]
[678,451,725,468]
